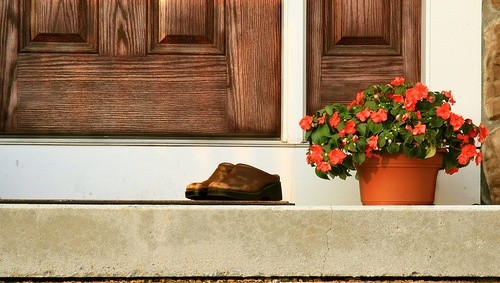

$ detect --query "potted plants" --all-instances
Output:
[355,142,440,204]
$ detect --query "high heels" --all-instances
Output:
[211,163,284,200]
[185,161,234,198]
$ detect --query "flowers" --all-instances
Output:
[298,79,490,184]
